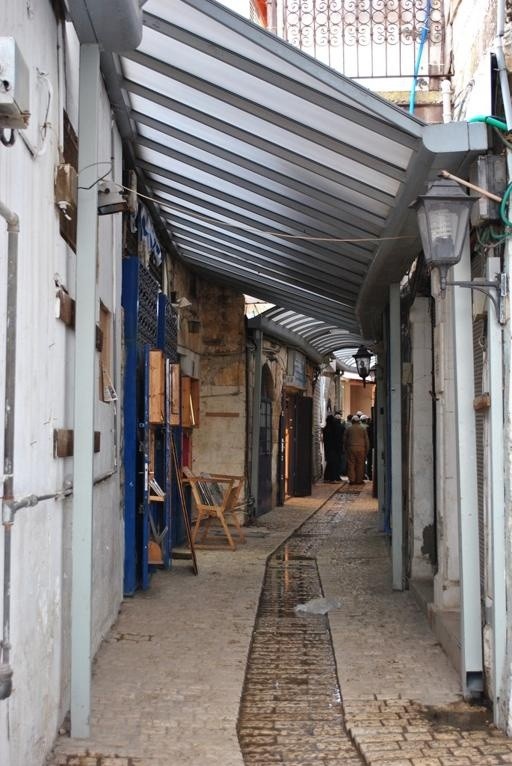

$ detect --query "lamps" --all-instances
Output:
[352,344,378,391]
[408,179,503,317]
[94,177,131,216]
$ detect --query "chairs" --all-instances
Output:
[181,464,247,554]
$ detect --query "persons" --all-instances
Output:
[323,410,373,485]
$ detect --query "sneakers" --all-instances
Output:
[349,480,365,485]
[324,480,344,484]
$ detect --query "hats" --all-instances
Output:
[352,411,370,422]
[335,415,343,420]
